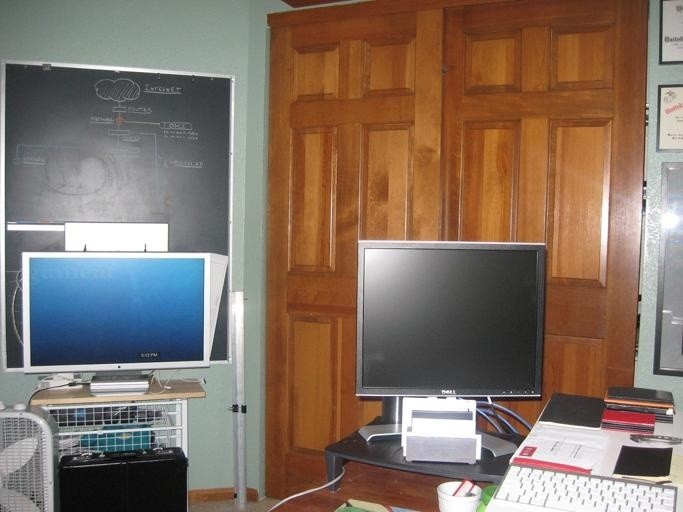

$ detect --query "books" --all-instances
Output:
[509,385,676,477]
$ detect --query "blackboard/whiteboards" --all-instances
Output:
[1,59,236,375]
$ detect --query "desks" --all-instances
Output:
[30,378,205,511]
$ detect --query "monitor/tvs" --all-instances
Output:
[355,239,546,459]
[20,249,212,393]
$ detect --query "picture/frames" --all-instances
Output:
[653,2,683,379]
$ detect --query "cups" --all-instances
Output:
[437,481,482,512]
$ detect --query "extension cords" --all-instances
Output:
[39,380,83,391]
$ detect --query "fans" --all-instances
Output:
[1,401,61,511]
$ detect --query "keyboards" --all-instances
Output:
[486,464,683,512]
